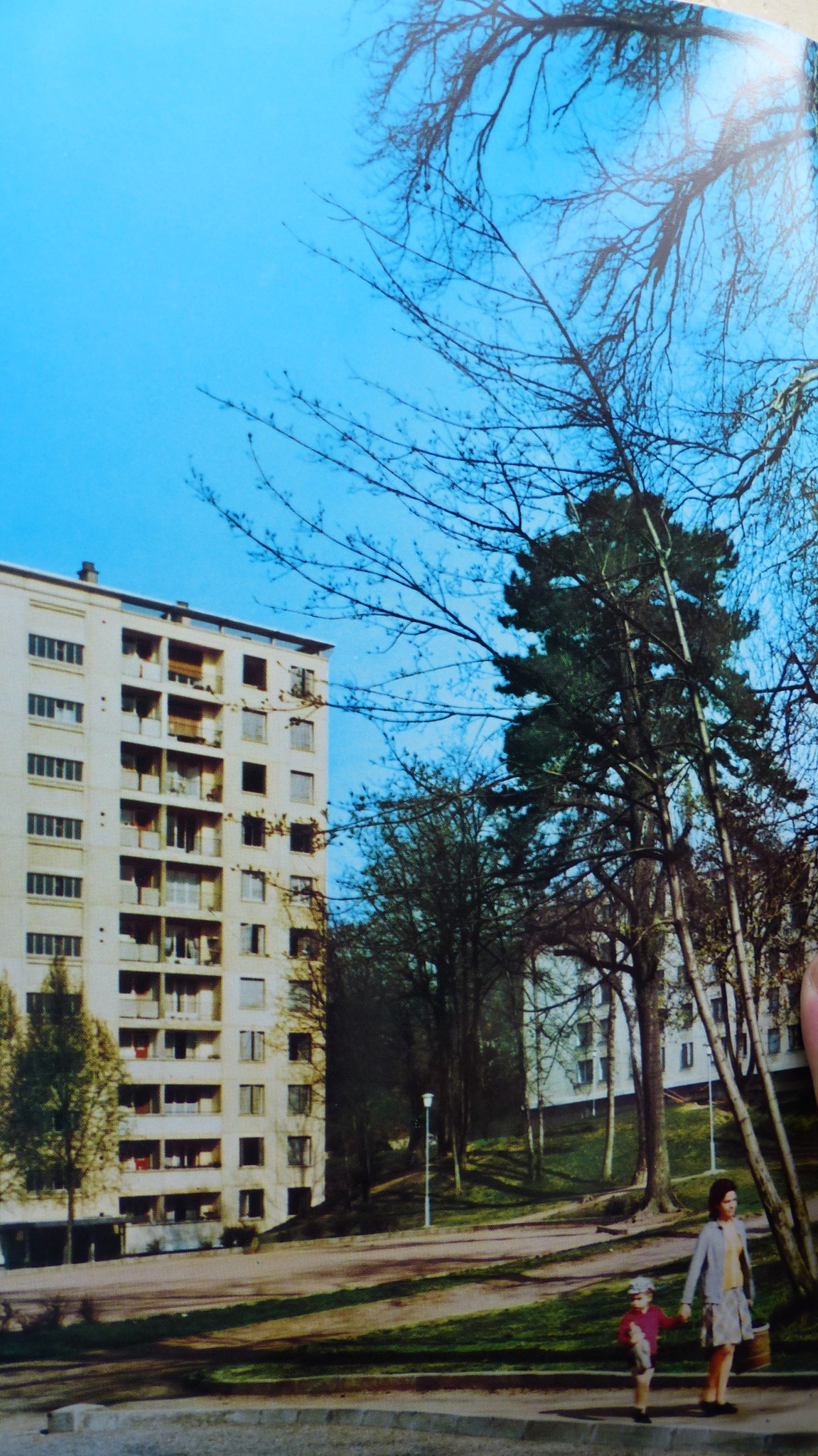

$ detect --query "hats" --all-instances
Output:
[626,1277,654,1294]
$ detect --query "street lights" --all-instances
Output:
[423,1093,435,1227]
[591,1050,598,1116]
[703,1042,716,1170]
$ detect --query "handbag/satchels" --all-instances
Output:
[731,1321,770,1373]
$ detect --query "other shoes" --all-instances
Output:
[716,1401,738,1415]
[700,1400,718,1417]
[634,1408,651,1424]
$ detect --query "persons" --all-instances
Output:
[677,1179,755,1415]
[618,1277,680,1424]
